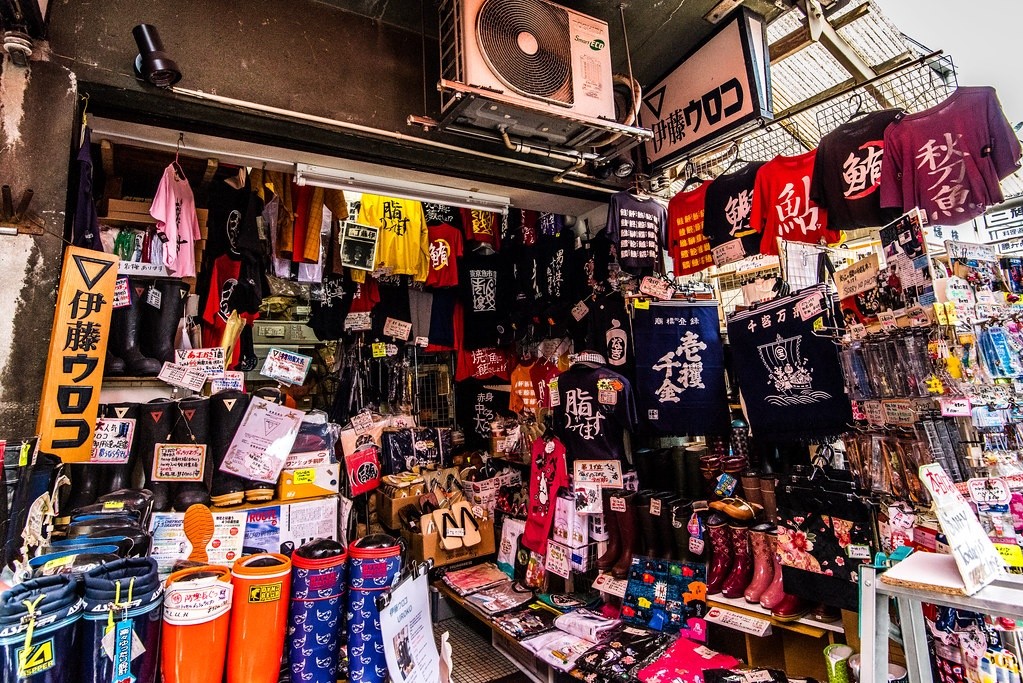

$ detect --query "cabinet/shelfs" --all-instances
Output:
[707,600,838,682]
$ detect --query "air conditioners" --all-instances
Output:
[437,1,616,151]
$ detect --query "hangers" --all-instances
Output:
[627,175,651,201]
[642,266,871,510]
[721,144,751,175]
[671,162,706,204]
[900,63,958,116]
[778,122,820,155]
[839,93,875,135]
[81,95,88,143]
[171,138,188,181]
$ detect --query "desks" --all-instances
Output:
[147,492,339,583]
[432,581,591,682]
[873,550,1023,683]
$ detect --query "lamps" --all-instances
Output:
[131,22,182,89]
[594,153,634,182]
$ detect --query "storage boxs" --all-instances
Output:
[400,519,496,568]
[276,461,342,501]
[374,488,424,530]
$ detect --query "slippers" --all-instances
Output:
[379,448,514,551]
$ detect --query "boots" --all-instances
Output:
[1,279,405,683]
[593,445,865,623]
